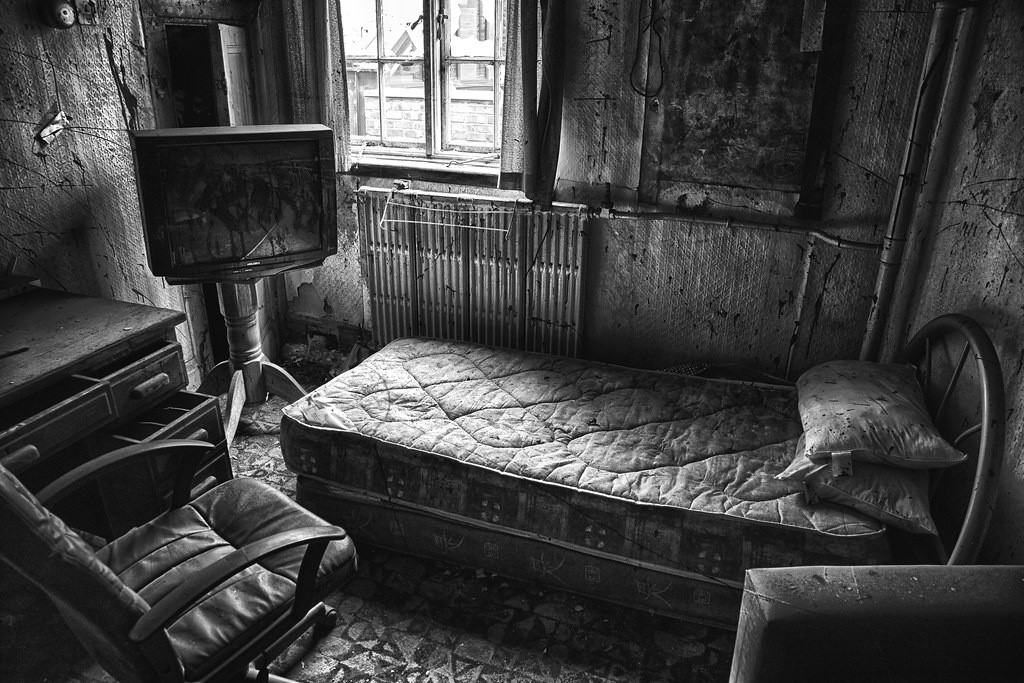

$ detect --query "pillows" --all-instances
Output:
[774,433,939,535]
[795,359,970,463]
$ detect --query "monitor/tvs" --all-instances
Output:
[126,124,337,286]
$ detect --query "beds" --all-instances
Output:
[279,311,1007,628]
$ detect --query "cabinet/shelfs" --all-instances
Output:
[3,293,233,545]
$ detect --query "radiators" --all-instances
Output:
[359,185,589,359]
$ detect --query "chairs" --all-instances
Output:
[1,459,358,682]
